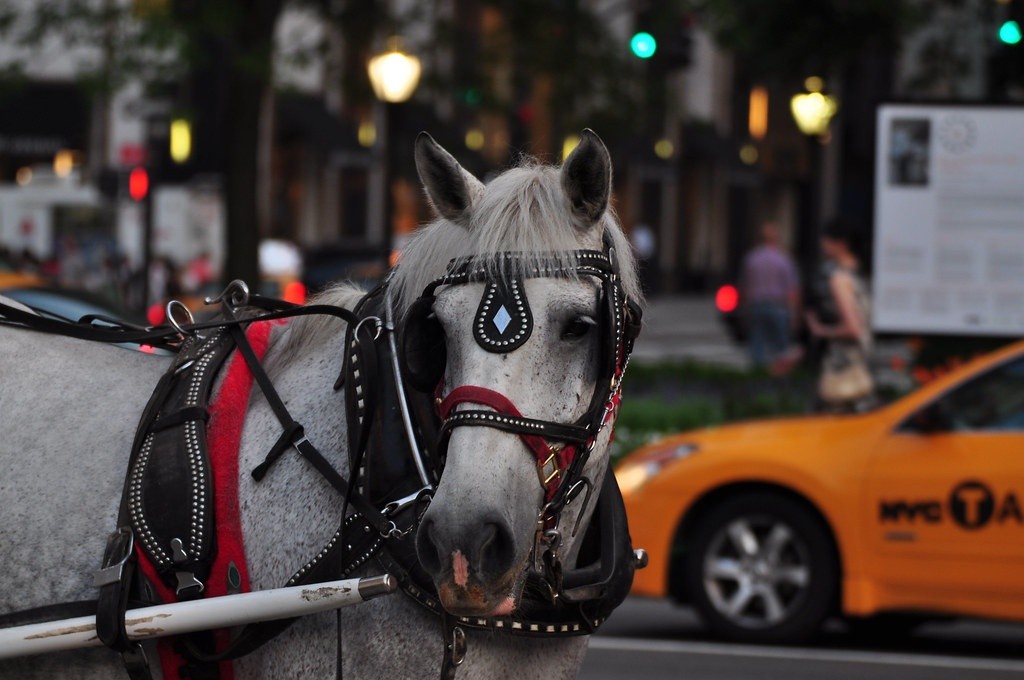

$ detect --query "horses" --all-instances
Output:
[0,128,644,679]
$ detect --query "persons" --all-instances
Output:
[8,220,218,318]
[807,218,876,416]
[631,222,656,295]
[747,222,799,369]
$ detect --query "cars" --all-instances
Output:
[610,339,1024,639]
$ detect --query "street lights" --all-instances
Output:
[782,73,837,329]
[366,36,421,264]
